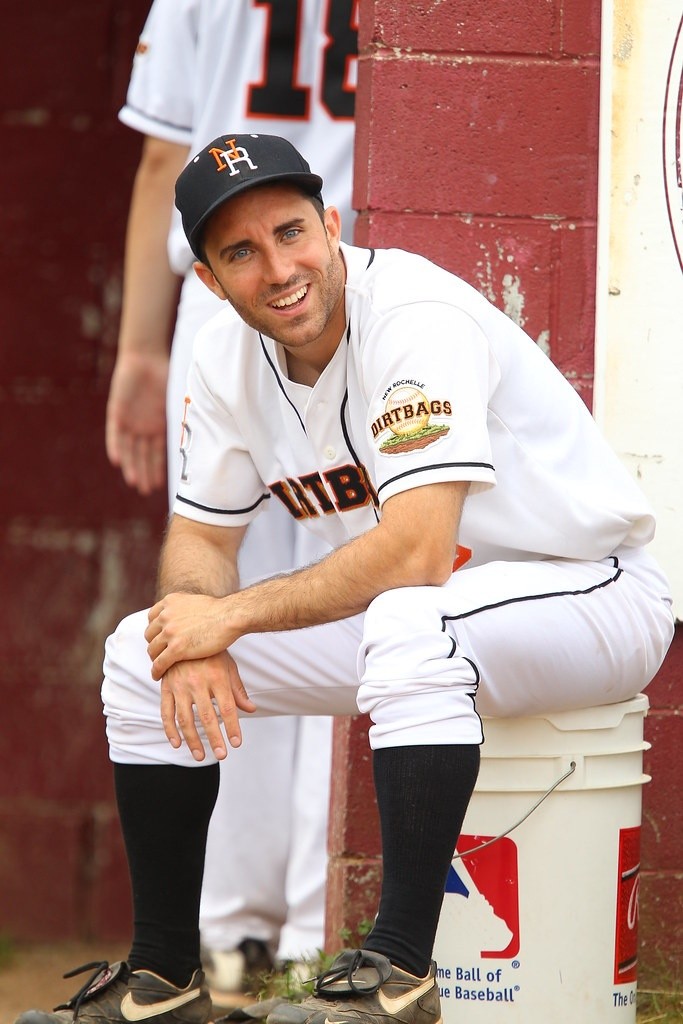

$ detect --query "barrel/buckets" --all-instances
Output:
[431,693,649,1024]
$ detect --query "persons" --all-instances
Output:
[12,133,674,1024]
[105,0,359,996]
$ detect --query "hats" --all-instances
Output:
[174,134,324,263]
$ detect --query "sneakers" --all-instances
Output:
[301,948,443,1024]
[15,960,212,1024]
